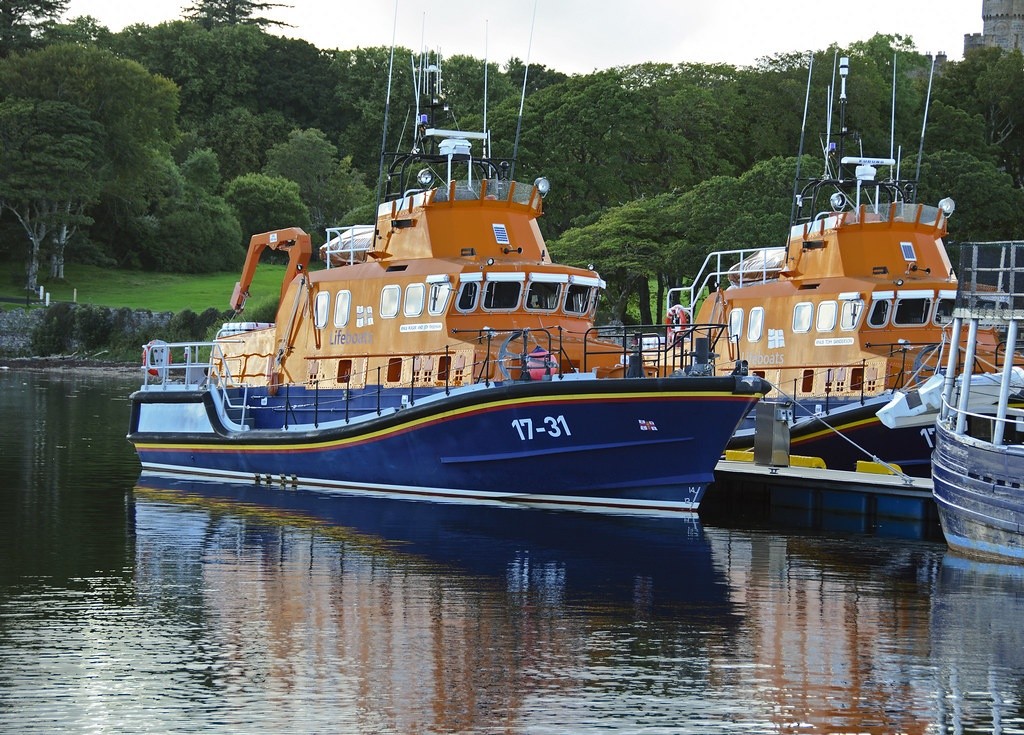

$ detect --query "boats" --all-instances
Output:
[124,2,774,545]
[626,43,1024,544]
[130,467,740,620]
[871,240,1024,566]
[876,366,1024,429]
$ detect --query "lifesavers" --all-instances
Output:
[143,339,174,374]
[666,305,686,334]
